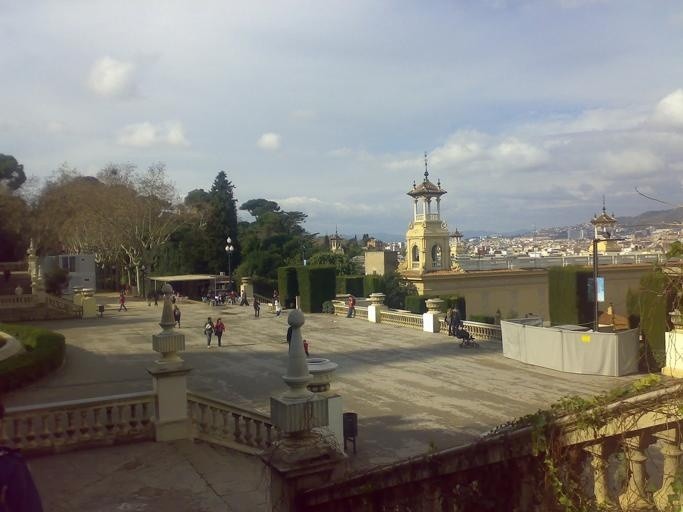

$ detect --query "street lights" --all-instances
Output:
[225,237,234,283]
[142,265,145,298]
[593,232,625,331]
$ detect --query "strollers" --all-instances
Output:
[456,328,479,349]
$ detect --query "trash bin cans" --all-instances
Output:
[99,306,104,317]
[343,412,358,454]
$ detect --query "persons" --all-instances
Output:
[493,308,500,324]
[274,295,281,318]
[0,402,43,512]
[200,289,249,307]
[445,304,452,336]
[345,295,355,318]
[450,303,460,336]
[173,305,180,328]
[252,298,260,317]
[272,287,277,303]
[153,291,157,305]
[213,318,225,347]
[146,293,152,306]
[202,316,214,349]
[303,339,309,357]
[606,302,614,324]
[286,326,292,353]
[117,293,126,311]
